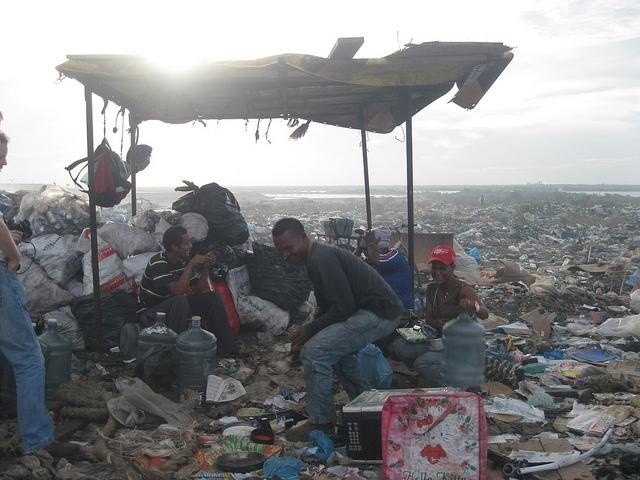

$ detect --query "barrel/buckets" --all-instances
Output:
[39,319,72,397]
[135,312,177,390]
[1,322,37,417]
[341,346,398,402]
[176,316,218,394]
[442,309,488,390]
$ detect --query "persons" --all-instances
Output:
[0,128,85,459]
[353,226,413,350]
[386,243,489,361]
[139,226,248,357]
[271,217,404,443]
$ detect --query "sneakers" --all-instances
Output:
[285,421,336,442]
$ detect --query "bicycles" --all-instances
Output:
[317,212,419,299]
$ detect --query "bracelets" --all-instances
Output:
[475,301,479,313]
[367,241,375,247]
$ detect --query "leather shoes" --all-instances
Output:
[40,438,83,458]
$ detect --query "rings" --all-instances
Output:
[467,300,471,304]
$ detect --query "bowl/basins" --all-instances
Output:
[215,426,274,473]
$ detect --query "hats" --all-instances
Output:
[357,226,392,250]
[427,244,456,267]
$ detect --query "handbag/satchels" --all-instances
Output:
[380,391,489,480]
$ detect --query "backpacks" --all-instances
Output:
[65,139,132,208]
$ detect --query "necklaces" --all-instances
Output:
[436,289,449,305]
